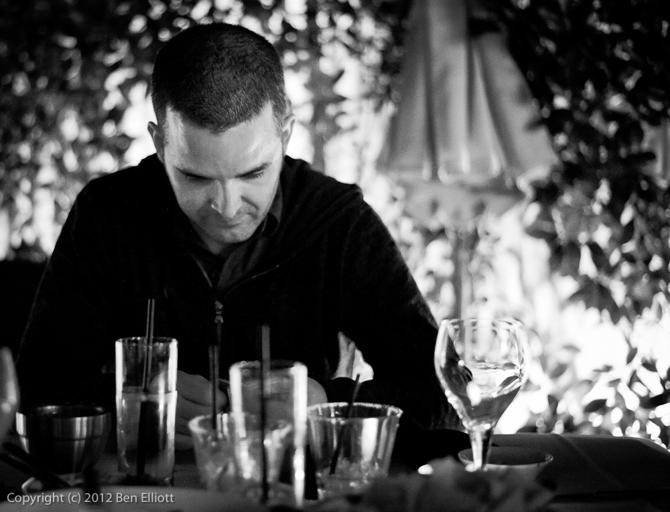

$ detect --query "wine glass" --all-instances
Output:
[436,317,527,472]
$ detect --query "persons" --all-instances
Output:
[15,22,474,463]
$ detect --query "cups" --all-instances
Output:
[114,337,405,502]
[457,446,554,483]
[15,405,111,474]
